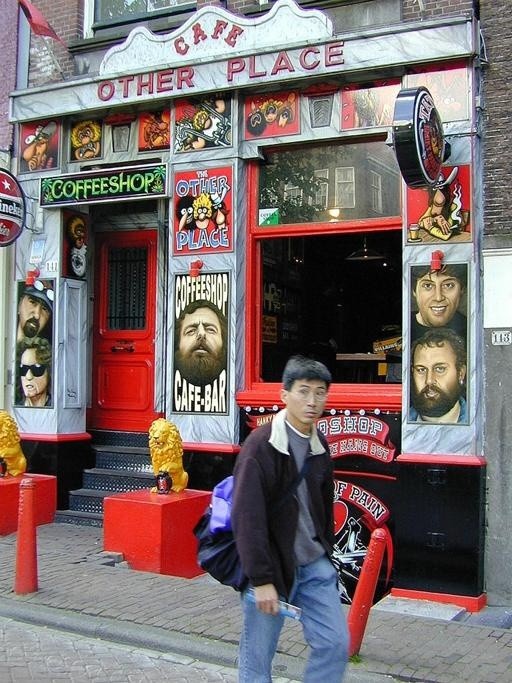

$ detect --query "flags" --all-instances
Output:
[19,0,70,54]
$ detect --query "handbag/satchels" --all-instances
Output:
[193,476,249,592]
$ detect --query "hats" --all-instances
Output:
[23,280,53,311]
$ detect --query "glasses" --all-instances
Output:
[33,280,55,302]
[18,364,47,377]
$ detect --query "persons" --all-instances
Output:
[408,328,467,424]
[230,358,352,683]
[16,336,52,408]
[410,263,467,341]
[174,300,229,387]
[16,280,55,341]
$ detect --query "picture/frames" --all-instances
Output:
[289,236,305,261]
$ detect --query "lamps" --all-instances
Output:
[345,234,386,261]
[289,257,304,264]
